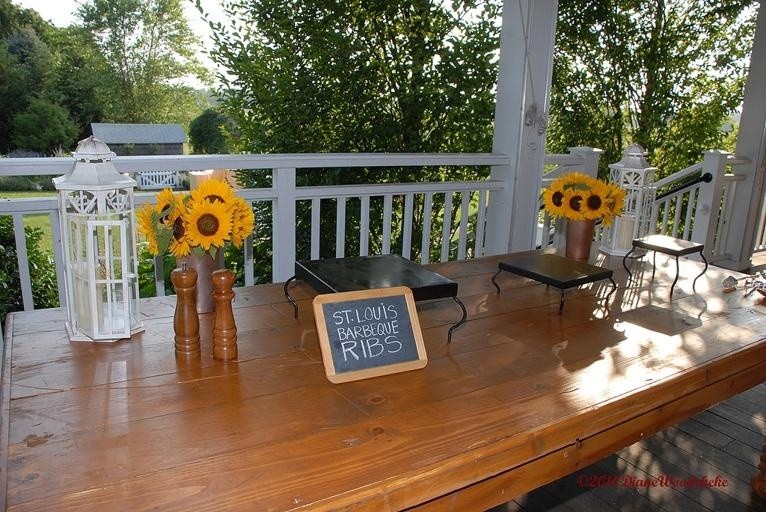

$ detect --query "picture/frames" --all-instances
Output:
[313,286,428,383]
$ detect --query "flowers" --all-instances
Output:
[135,176,256,257]
[539,172,626,229]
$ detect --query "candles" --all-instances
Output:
[617,214,634,250]
[75,269,104,330]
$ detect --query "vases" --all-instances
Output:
[567,217,596,259]
[175,246,224,314]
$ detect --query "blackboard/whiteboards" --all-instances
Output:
[318,295,421,375]
